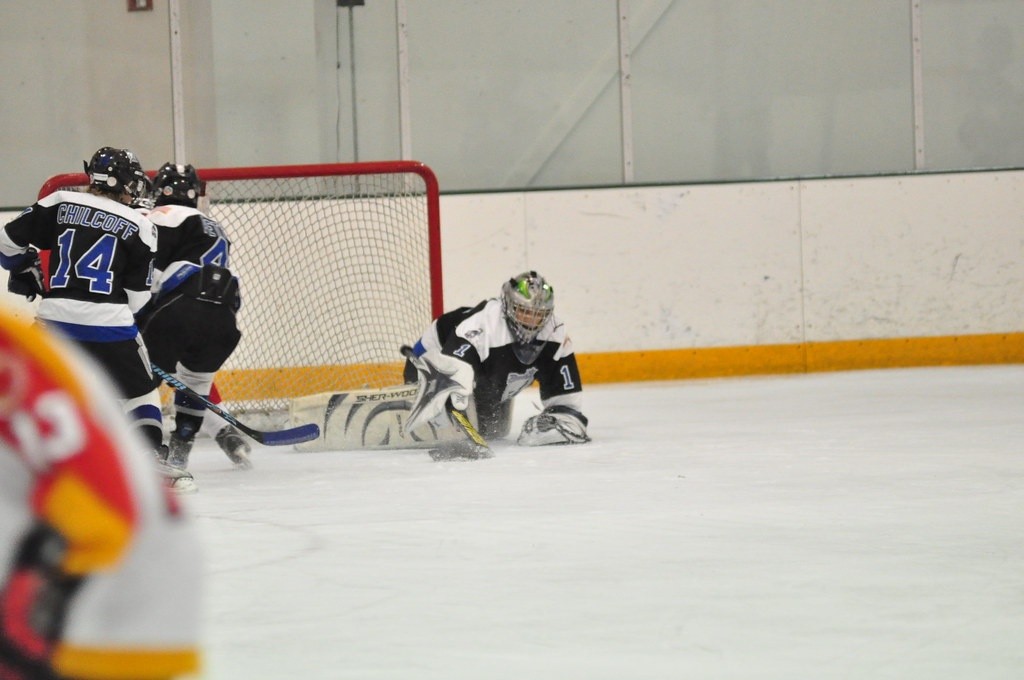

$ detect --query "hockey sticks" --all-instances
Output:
[150,364,319,445]
[400,343,496,462]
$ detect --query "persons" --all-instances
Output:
[276,271,591,460]
[0,292,208,680]
[0,146,252,493]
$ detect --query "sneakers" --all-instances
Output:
[169,431,194,471]
[215,424,253,468]
[150,444,194,495]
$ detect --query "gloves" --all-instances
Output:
[8,252,45,302]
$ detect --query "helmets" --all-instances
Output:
[154,162,199,208]
[502,271,554,346]
[84,147,153,205]
